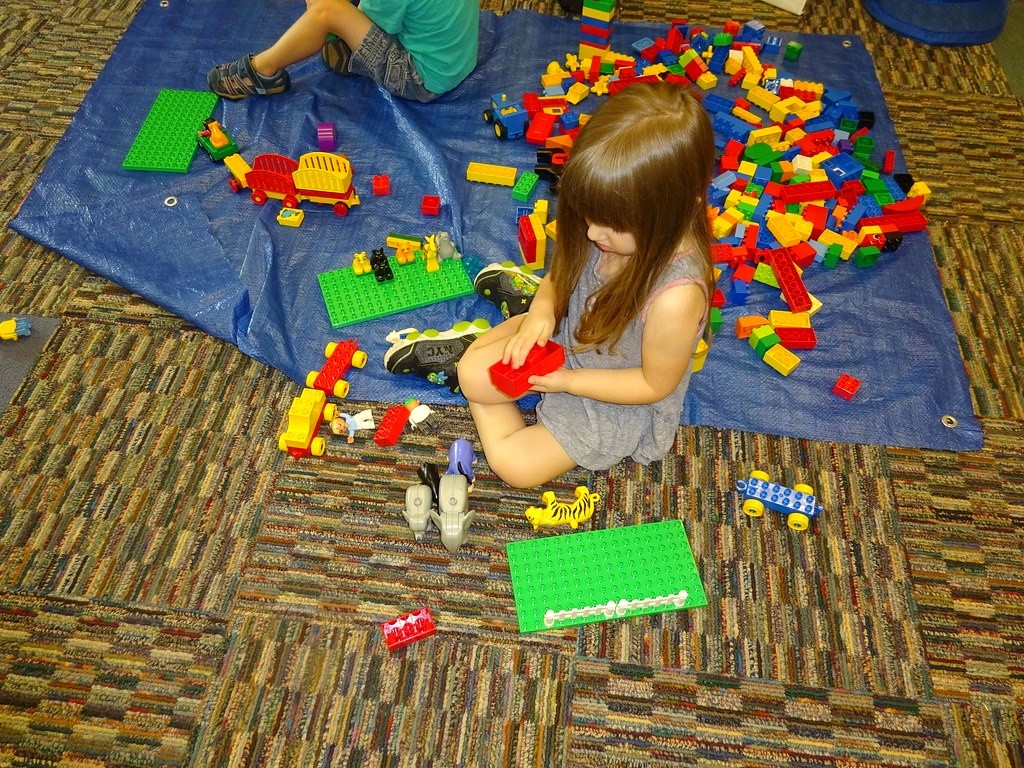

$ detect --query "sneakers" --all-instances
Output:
[208,54,290,98]
[318,38,355,78]
[474,260,544,320]
[384,318,493,394]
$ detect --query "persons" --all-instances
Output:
[330,409,383,443]
[384,82,715,488]
[207,0,480,102]
[403,398,439,436]
[0,317,31,341]
